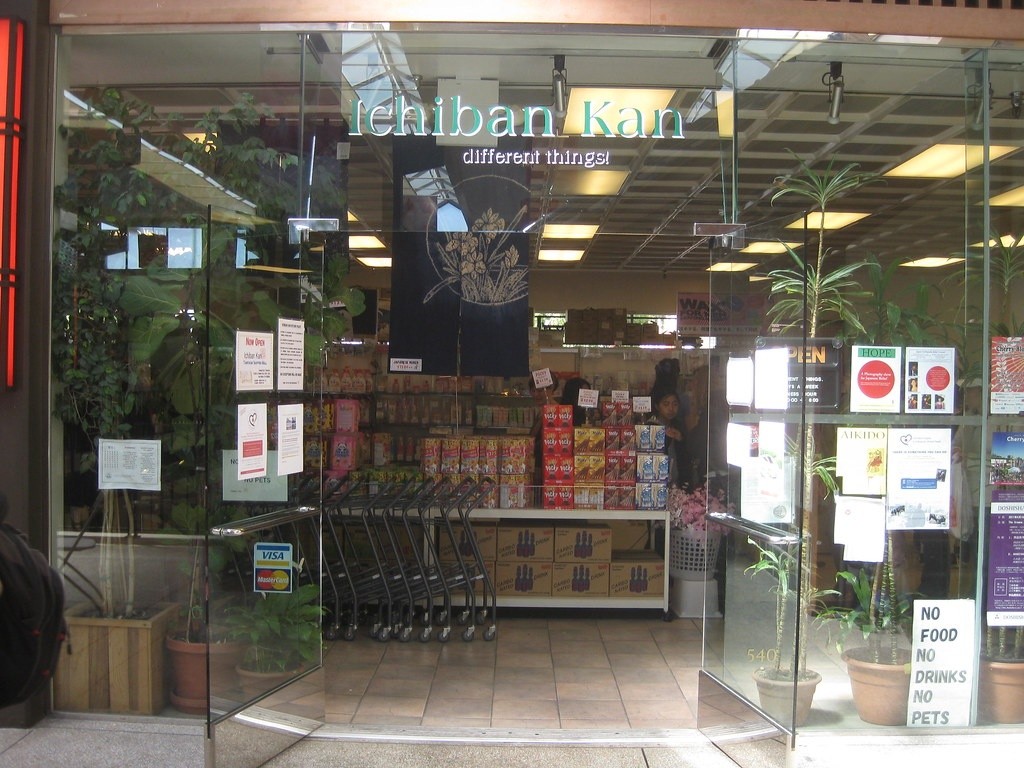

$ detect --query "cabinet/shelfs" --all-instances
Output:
[270,391,563,471]
[236,509,670,621]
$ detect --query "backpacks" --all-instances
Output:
[0,525,65,708]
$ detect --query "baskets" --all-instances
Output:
[670,528,723,580]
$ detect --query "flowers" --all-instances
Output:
[664,478,737,536]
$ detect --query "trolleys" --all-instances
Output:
[229,468,495,643]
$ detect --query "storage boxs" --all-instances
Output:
[438,521,664,596]
[310,520,422,563]
[566,308,676,345]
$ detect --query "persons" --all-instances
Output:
[650,359,680,396]
[528,369,559,404]
[653,392,687,557]
[665,366,728,494]
[561,377,601,424]
[907,384,964,609]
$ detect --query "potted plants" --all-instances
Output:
[50,84,366,723]
[752,135,1023,725]
